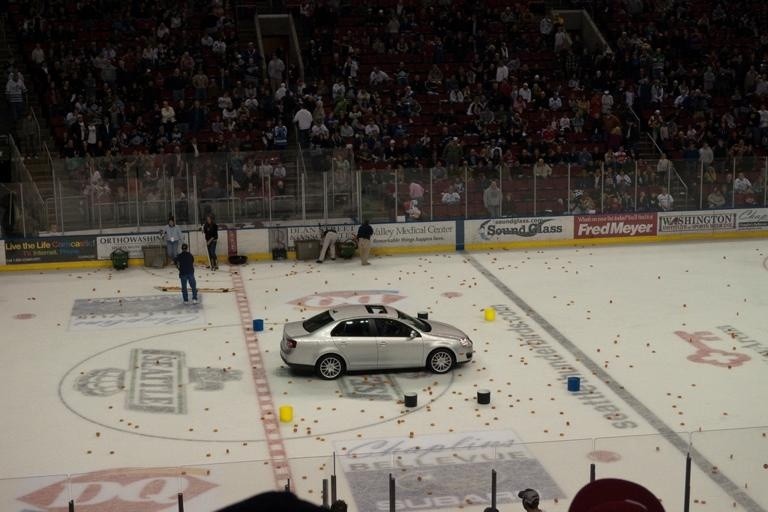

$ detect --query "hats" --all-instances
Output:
[518,489,539,505]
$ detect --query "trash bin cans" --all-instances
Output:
[294,239,321,261]
[142,245,168,266]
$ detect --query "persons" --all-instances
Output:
[357,219,373,265]
[332,499,346,512]
[518,488,545,511]
[211,488,330,511]
[316,230,338,263]
[1,1,768,232]
[175,244,198,305]
[162,219,182,264]
[203,214,218,269]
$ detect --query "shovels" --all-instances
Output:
[272,224,286,260]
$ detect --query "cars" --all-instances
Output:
[280,304,473,380]
